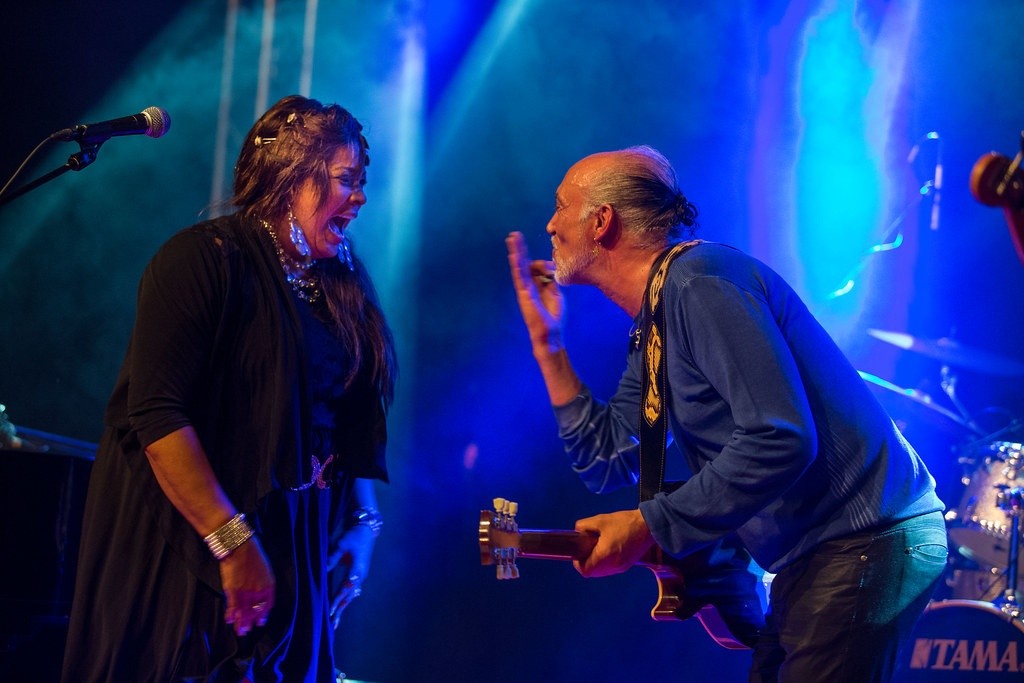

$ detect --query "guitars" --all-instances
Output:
[476,479,773,650]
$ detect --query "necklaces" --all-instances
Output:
[628,290,646,349]
[257,216,322,304]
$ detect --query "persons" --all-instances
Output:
[506,146,949,683]
[64,93,401,683]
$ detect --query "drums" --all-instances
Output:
[947,439,1023,580]
[950,567,1010,604]
[881,600,1024,683]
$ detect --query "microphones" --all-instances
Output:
[48,105,171,145]
[968,151,1024,208]
[930,138,945,229]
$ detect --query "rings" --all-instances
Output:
[354,588,362,597]
[342,579,354,589]
[253,602,271,612]
[348,572,359,581]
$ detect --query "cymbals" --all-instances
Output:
[856,370,988,433]
[866,329,1022,385]
[2,418,99,462]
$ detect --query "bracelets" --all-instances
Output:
[349,505,383,534]
[204,513,255,559]
